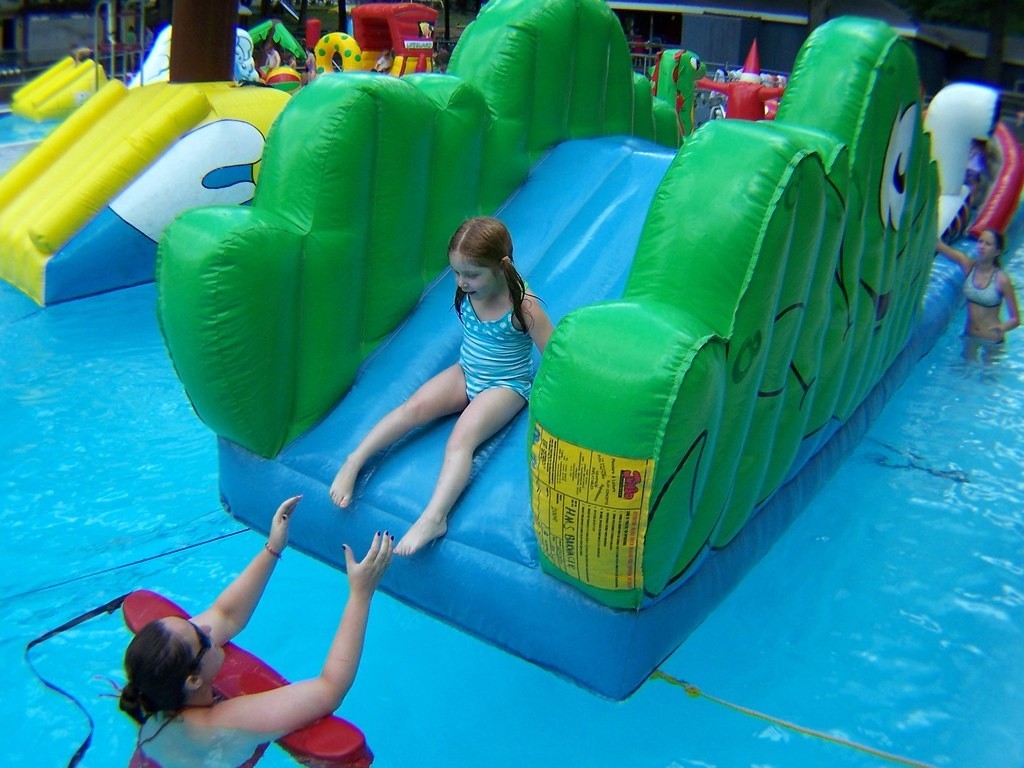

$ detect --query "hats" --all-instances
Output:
[264,44,271,50]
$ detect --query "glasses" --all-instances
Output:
[189,621,211,670]
[439,68,446,72]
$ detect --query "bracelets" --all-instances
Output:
[264,542,282,559]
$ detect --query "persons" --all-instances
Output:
[935,227,1022,364]
[328,217,554,558]
[121,495,394,768]
[433,44,451,73]
[254,40,315,85]
[373,49,394,74]
